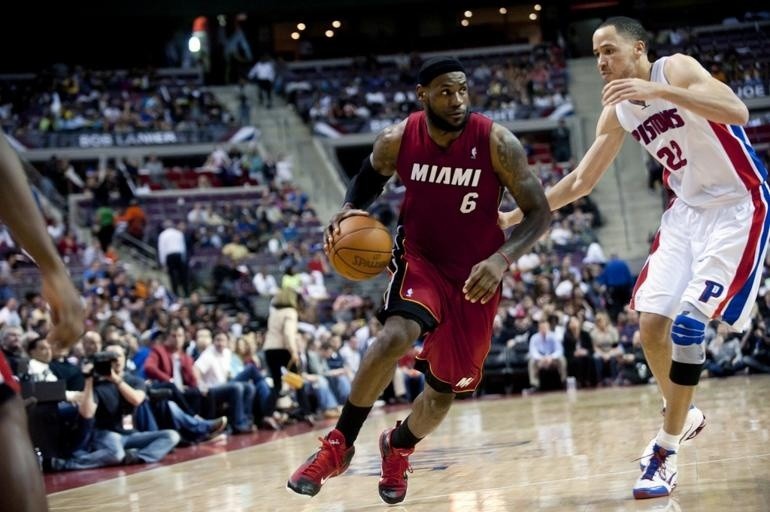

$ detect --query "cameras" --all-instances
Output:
[88,351,118,376]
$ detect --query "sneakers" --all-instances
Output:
[231,411,297,434]
[287,429,355,496]
[397,395,409,404]
[303,415,316,426]
[108,446,142,467]
[324,404,345,418]
[699,369,713,379]
[376,427,416,507]
[633,404,706,501]
[202,415,229,441]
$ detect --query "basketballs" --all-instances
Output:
[328,215,392,282]
